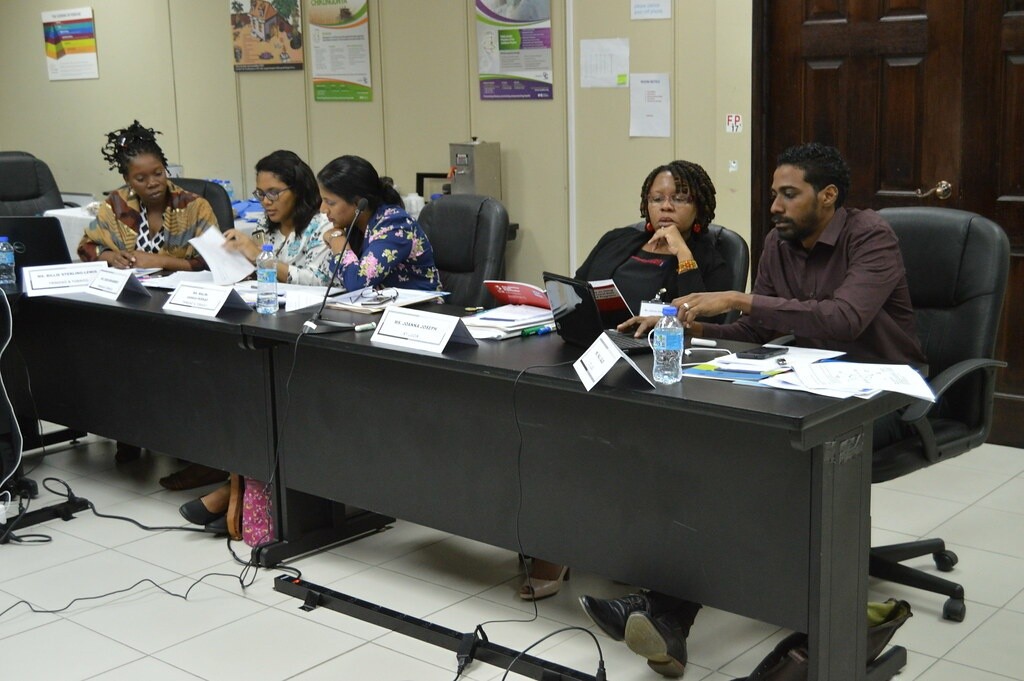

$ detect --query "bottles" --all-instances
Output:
[652,307,684,384]
[256,245,279,314]
[0,236,15,286]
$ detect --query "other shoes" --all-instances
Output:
[159,463,230,489]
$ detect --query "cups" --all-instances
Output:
[402,192,425,220]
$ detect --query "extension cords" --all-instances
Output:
[273,574,609,681]
[0,496,88,531]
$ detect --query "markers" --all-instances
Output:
[538,326,556,335]
[522,323,554,337]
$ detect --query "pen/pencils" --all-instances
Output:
[480,317,515,321]
[117,239,133,268]
[233,283,257,289]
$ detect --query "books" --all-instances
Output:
[484,280,634,328]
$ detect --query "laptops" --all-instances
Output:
[0,216,73,270]
[543,271,653,354]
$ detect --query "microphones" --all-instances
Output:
[301,198,368,334]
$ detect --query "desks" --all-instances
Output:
[0,278,285,568]
[242,301,929,681]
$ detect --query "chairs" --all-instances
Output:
[870,207,1010,621]
[0,151,65,216]
[419,194,507,307]
[168,179,236,241]
[626,222,750,328]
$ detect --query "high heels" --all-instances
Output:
[520,562,571,599]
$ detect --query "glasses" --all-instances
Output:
[253,188,289,201]
[350,288,399,304]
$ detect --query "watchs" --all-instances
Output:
[327,230,346,243]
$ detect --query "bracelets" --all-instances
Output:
[676,260,698,272]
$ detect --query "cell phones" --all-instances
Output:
[737,347,789,359]
[361,297,392,305]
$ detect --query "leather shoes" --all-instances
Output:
[578,591,652,639]
[180,495,228,525]
[203,514,244,534]
[626,610,687,678]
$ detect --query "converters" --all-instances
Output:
[456,633,479,664]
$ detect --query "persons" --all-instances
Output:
[577,140,930,677]
[159,150,341,490]
[77,119,219,462]
[485,160,731,603]
[178,154,447,537]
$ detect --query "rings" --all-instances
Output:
[684,303,691,311]
[661,227,665,230]
